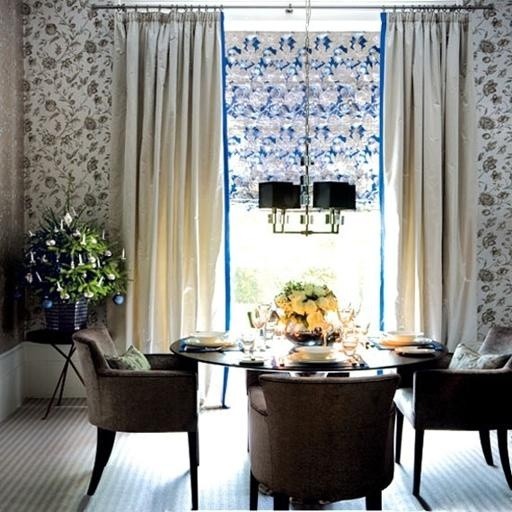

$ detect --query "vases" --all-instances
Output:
[41,289,91,332]
[286,327,343,347]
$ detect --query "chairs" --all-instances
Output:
[72,321,200,510]
[392,323,511,495]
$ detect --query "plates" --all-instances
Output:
[288,352,344,365]
[381,337,433,347]
[184,338,234,348]
[395,348,435,355]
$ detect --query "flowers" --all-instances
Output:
[22,203,132,306]
[272,278,341,337]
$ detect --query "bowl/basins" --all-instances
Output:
[192,334,218,346]
[396,334,415,343]
[296,346,332,362]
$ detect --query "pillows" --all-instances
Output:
[106,343,151,371]
[448,342,510,373]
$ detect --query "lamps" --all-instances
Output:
[258,7,357,237]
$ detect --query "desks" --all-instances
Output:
[27,330,114,419]
[169,325,452,373]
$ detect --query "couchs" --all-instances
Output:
[244,373,401,511]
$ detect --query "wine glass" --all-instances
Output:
[354,321,370,353]
[337,308,355,341]
[249,309,269,348]
[348,301,361,337]
[253,296,275,349]
[343,336,358,366]
[239,330,255,361]
